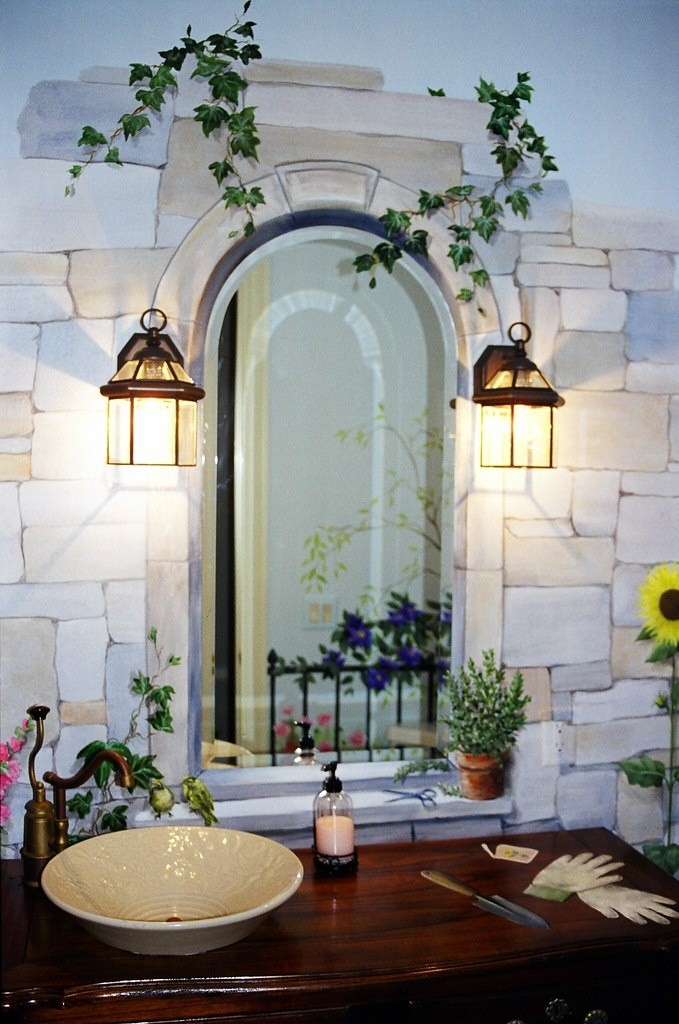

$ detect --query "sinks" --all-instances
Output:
[41,825,301,957]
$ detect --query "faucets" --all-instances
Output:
[42,750,135,850]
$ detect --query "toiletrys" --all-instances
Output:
[311,763,355,881]
[292,721,320,767]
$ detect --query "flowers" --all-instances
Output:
[611,564,678,879]
[268,589,453,753]
[0,715,35,835]
[0,710,36,857]
[269,591,454,761]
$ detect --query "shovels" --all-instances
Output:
[420,867,549,930]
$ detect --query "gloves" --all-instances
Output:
[523,851,679,926]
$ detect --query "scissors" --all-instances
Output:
[383,788,437,806]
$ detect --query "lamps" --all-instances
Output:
[100,309,205,466]
[473,323,566,469]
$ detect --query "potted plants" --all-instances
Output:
[393,647,535,802]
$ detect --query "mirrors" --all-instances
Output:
[184,208,471,804]
